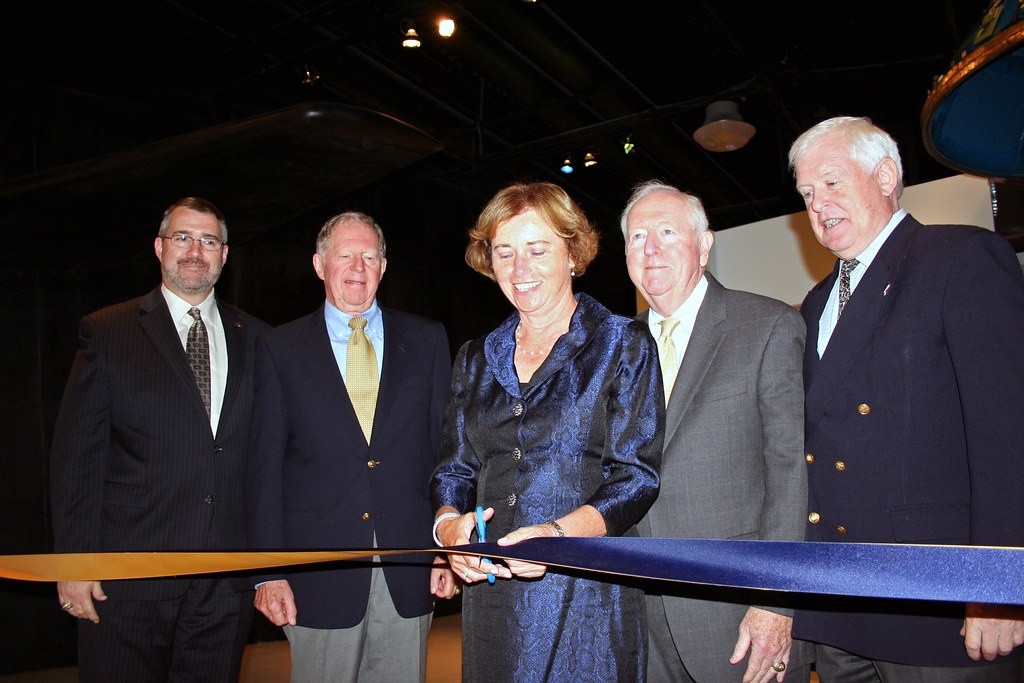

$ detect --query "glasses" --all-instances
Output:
[160,232,225,251]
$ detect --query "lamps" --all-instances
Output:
[399,17,421,49]
[693,100,757,152]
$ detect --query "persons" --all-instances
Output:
[248,212,453,683]
[51,196,277,683]
[784,116,1024,683]
[621,183,809,683]
[429,182,667,683]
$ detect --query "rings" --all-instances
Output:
[771,661,786,672]
[462,568,469,579]
[62,602,73,610]
[455,587,462,595]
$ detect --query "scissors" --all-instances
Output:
[472,506,496,584]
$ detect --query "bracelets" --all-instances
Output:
[546,520,564,537]
[432,513,459,548]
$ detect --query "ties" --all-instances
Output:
[838,258,860,320]
[347,317,379,445]
[187,307,211,422]
[657,318,681,410]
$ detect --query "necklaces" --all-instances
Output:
[516,320,569,356]
